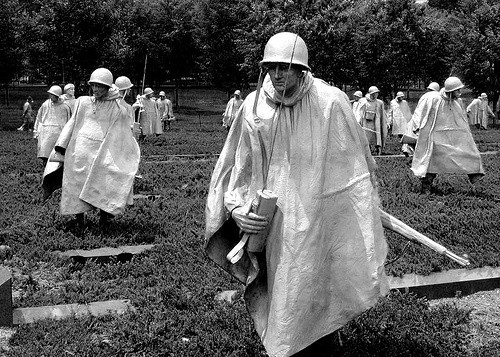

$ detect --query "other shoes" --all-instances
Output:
[17,127,24,131]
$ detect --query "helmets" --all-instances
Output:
[64,83,75,93]
[47,85,62,97]
[481,93,487,98]
[397,92,405,97]
[369,86,380,95]
[144,88,154,95]
[159,91,166,97]
[444,78,464,92]
[354,91,362,97]
[115,76,133,91]
[427,82,440,91]
[88,68,113,88]
[234,90,240,95]
[259,31,312,71]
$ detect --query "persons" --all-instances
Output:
[41,68,140,232]
[17,68,176,232]
[204,32,389,357]
[387,91,411,135]
[221,89,243,131]
[410,76,485,197]
[398,82,440,158]
[466,92,495,131]
[354,86,390,159]
[349,90,363,106]
